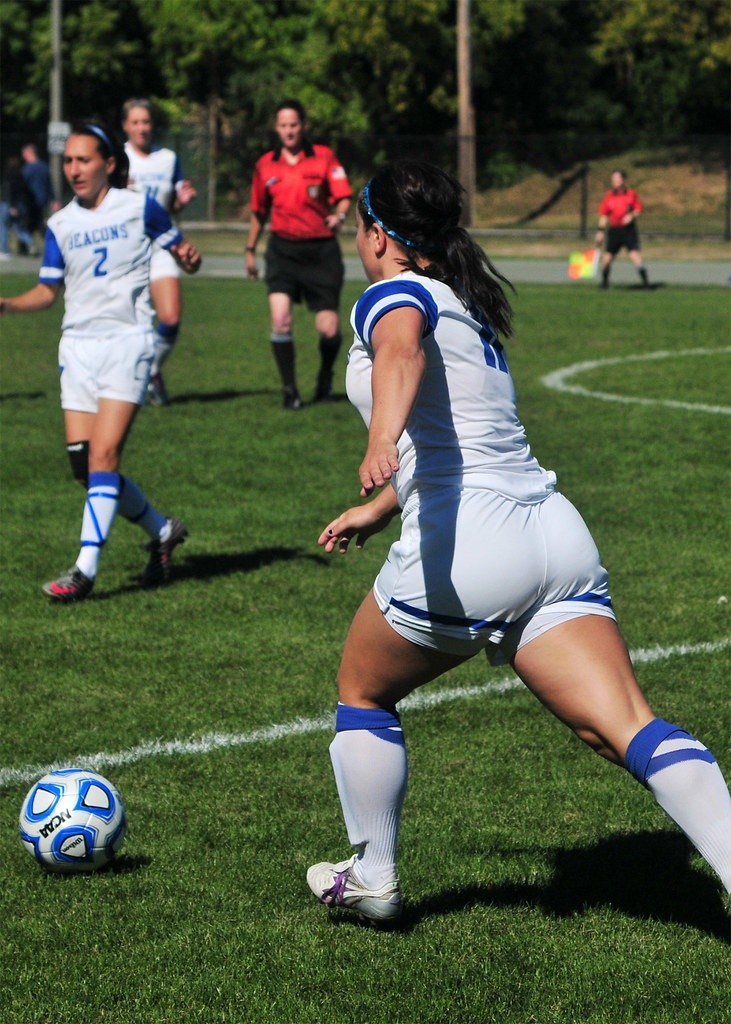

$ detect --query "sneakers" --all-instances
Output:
[307,854,399,920]
[146,371,166,408]
[145,517,187,578]
[42,566,94,601]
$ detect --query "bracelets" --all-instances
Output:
[597,227,605,230]
[335,210,346,221]
[245,247,255,252]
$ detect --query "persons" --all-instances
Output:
[17,142,55,255]
[113,98,197,404]
[595,169,653,289]
[0,142,38,262]
[308,159,731,924]
[0,119,202,602]
[245,99,352,408]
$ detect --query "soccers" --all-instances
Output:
[19,765,128,873]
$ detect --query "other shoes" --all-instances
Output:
[313,377,333,404]
[283,387,303,411]
